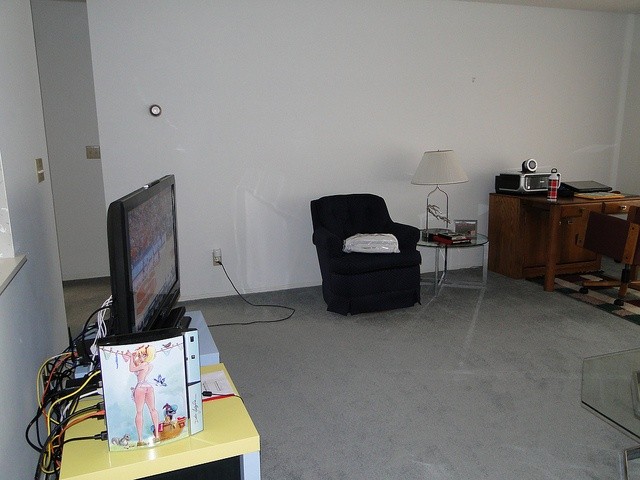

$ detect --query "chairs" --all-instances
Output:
[574,204,639,306]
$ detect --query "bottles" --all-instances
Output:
[547,167,559,201]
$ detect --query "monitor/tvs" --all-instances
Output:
[108,173,192,335]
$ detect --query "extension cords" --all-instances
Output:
[67,325,100,379]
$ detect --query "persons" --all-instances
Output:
[124,344,160,445]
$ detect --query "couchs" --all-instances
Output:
[309,193,422,316]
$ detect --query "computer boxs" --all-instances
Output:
[96,327,205,451]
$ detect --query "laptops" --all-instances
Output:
[561,181,612,193]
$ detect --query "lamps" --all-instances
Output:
[410,148,470,233]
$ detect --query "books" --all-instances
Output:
[573,190,624,200]
[200,371,234,400]
[435,232,471,244]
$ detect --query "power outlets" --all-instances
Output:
[86,145,100,159]
[36,159,43,171]
[213,248,222,266]
[37,172,44,183]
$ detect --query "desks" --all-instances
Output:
[53,360,264,480]
[67,307,223,381]
[579,348,639,480]
[487,190,640,282]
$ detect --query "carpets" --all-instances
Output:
[525,266,640,327]
